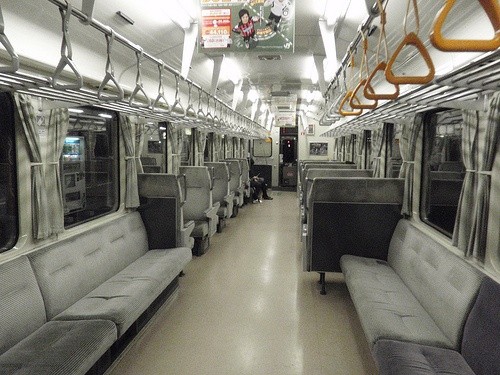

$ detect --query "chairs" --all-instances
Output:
[137,158,251,256]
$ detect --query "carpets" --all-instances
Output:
[272,186,296,192]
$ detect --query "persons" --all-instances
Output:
[231,9,261,48]
[247,158,273,204]
[263,0,294,31]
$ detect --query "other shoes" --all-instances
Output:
[262,193,273,200]
[253,192,259,201]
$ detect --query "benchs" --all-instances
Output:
[0,211,192,375]
[295,160,500,375]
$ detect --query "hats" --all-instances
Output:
[247,157,255,166]
